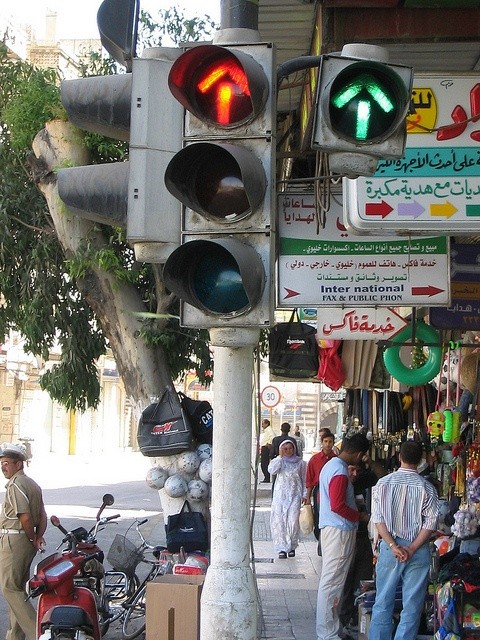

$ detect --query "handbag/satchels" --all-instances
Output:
[167,511,208,554]
[178,396,213,444]
[299,504,314,534]
[137,402,192,457]
[269,322,319,378]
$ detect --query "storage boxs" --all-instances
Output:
[146,572,207,640]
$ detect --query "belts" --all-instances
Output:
[366,391,373,467]
[425,383,437,414]
[354,388,361,439]
[0,529,24,534]
[383,390,407,461]
[362,389,368,429]
[422,386,427,428]
[413,388,420,433]
[407,387,415,441]
[378,392,383,459]
[373,390,378,461]
[343,388,354,426]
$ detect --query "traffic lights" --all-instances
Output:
[309,53,413,159]
[161,43,276,330]
[55,56,183,264]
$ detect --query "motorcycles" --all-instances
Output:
[22,493,120,640]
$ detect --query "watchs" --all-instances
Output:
[28,534,36,542]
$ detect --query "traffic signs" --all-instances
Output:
[261,385,282,408]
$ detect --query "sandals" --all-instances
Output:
[288,550,295,557]
[279,551,286,558]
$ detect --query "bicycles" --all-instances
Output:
[103,519,168,637]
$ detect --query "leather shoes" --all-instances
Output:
[261,479,270,483]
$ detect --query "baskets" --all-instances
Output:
[107,534,145,576]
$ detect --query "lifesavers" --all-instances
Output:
[384,321,446,387]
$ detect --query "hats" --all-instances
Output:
[0,442,26,461]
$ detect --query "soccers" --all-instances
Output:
[196,444,212,461]
[177,450,200,474]
[187,479,208,502]
[198,458,216,485]
[145,465,168,490]
[163,474,188,498]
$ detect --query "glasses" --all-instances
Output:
[1,461,16,466]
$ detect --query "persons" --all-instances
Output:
[367,439,440,640]
[266,438,310,559]
[314,432,373,640]
[270,422,301,502]
[319,427,341,457]
[0,442,48,640]
[289,425,306,460]
[335,452,391,635]
[304,432,339,557]
[259,419,276,483]
